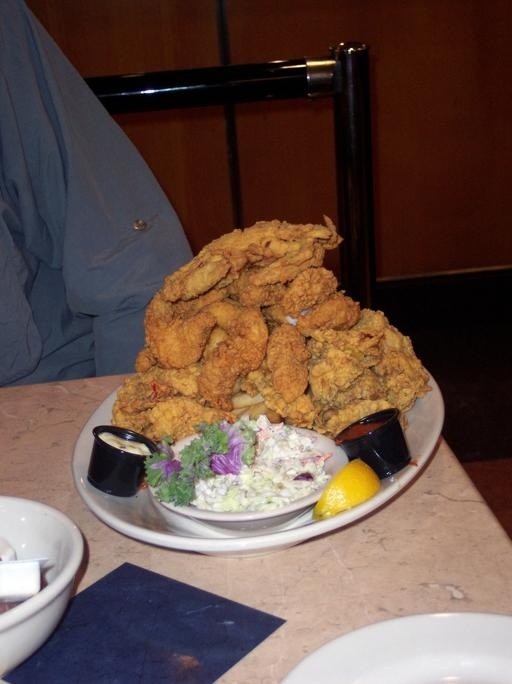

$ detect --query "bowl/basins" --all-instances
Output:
[0,494,85,679]
[144,419,353,535]
[70,362,449,561]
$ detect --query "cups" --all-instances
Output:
[336,408,412,480]
[86,425,160,498]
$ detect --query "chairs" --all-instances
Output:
[81,42,379,307]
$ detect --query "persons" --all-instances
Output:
[0,1,194,389]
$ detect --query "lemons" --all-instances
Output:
[312,458,381,524]
